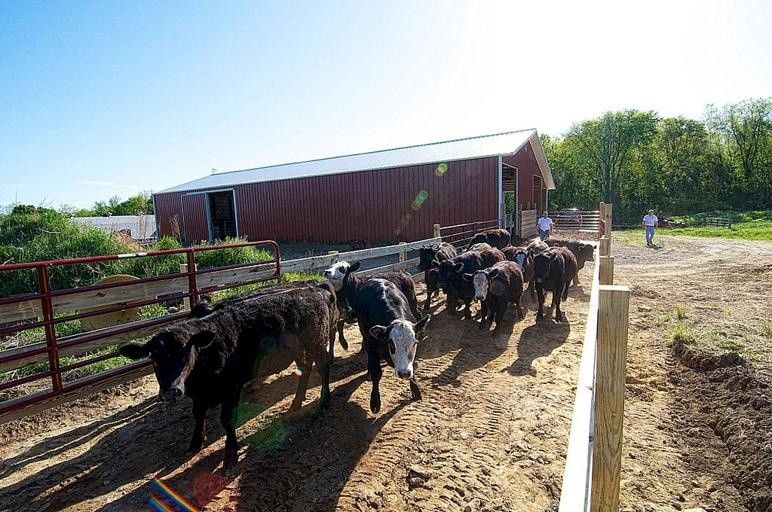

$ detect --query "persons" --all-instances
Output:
[536,211,553,241]
[642,209,658,246]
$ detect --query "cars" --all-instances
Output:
[554,207,583,230]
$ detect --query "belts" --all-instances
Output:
[646,226,654,227]
[541,230,549,231]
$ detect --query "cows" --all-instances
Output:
[187,278,350,396]
[415,227,598,331]
[117,285,336,476]
[321,259,427,351]
[354,275,429,416]
